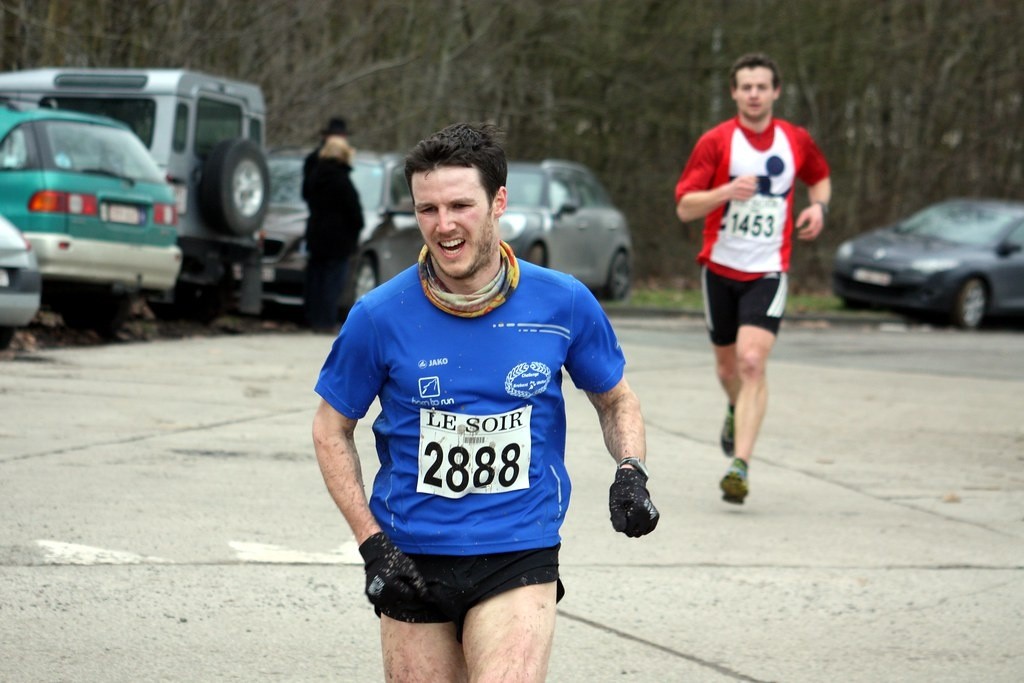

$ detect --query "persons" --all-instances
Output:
[301,115,365,335]
[674,52,831,505]
[311,124,661,683]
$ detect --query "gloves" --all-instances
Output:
[609,468,660,539]
[357,531,428,613]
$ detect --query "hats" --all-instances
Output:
[321,116,352,135]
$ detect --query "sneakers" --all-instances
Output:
[721,458,749,504]
[721,404,736,457]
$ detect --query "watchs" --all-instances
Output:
[617,456,649,482]
[811,200,828,213]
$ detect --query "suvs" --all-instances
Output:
[0,58,272,328]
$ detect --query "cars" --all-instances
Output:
[0,209,42,351]
[0,93,183,341]
[228,143,426,323]
[828,196,1024,333]
[500,156,634,301]
